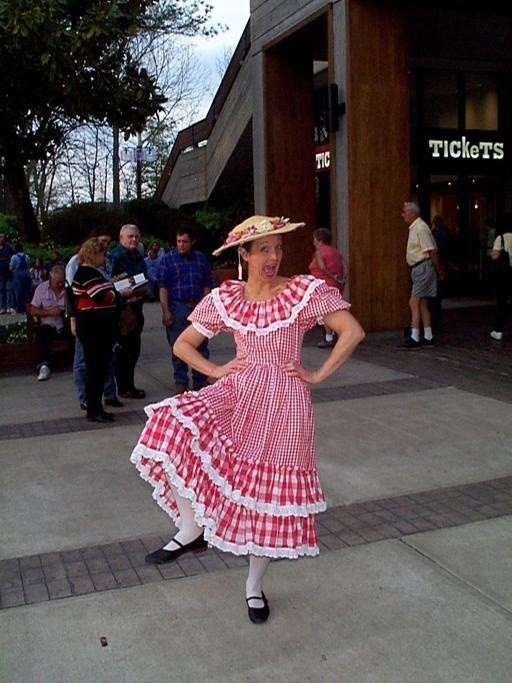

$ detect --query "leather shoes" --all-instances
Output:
[145,529,207,565]
[246,590,269,624]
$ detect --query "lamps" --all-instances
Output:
[323,82,346,132]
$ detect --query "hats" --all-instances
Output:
[213,215,305,256]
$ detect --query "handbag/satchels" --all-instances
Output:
[486,249,509,271]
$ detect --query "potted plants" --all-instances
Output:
[0,318,37,374]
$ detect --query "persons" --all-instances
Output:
[62,224,211,424]
[484,216,512,340]
[397,201,444,350]
[429,213,457,261]
[1,234,75,381]
[126,214,365,623]
[308,227,348,349]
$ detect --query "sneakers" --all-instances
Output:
[80,390,145,422]
[397,337,421,350]
[38,368,49,380]
[490,331,503,340]
[421,338,435,348]
[317,340,335,349]
